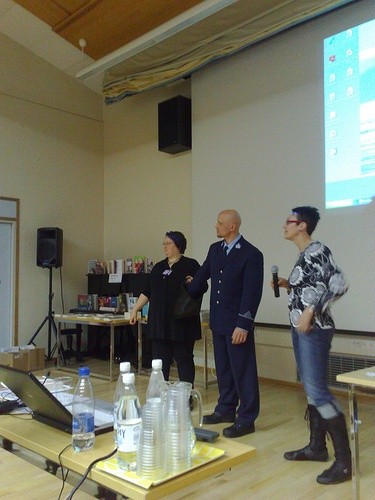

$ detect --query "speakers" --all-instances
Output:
[157,95,192,154]
[37,227,63,269]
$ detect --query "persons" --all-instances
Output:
[128,230,209,413]
[183,209,264,440]
[271,205,352,486]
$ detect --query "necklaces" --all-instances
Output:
[167,258,180,264]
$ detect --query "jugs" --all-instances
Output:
[160,380,204,458]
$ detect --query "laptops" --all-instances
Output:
[0,364,116,436]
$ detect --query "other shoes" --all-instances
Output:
[203,414,237,424]
[189,397,194,410]
[223,421,255,438]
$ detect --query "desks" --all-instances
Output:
[0,389,256,500]
[138,320,218,390]
[53,313,138,381]
[336,366,375,500]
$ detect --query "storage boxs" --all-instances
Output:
[0,347,45,372]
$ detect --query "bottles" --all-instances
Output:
[113,362,131,448]
[72,367,95,452]
[145,359,165,401]
[116,372,143,471]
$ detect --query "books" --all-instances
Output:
[102,259,126,275]
[77,292,150,317]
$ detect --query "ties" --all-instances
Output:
[223,246,228,255]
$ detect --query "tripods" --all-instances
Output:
[28,267,66,366]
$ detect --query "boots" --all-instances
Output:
[316,413,352,484]
[284,404,330,462]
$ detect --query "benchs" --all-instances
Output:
[0,447,99,500]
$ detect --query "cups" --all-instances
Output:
[37,376,73,404]
[137,386,192,480]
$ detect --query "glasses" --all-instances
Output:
[286,218,300,225]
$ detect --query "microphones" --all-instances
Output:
[271,265,280,298]
